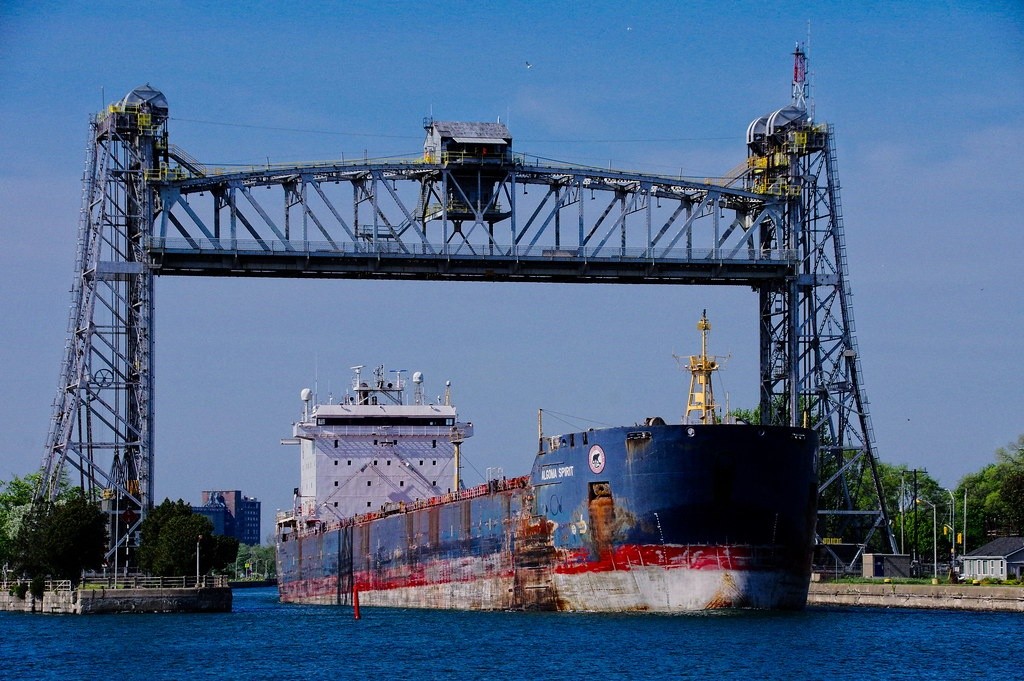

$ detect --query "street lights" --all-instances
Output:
[197,535,202,583]
[945,488,954,573]
[916,497,938,586]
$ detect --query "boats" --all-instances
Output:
[275,310,820,615]
